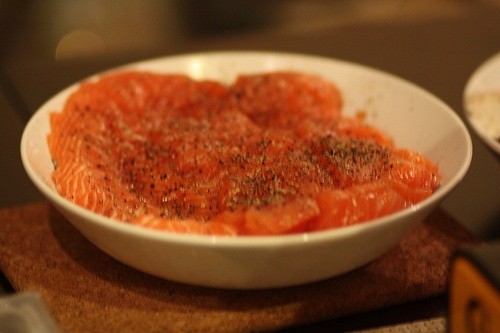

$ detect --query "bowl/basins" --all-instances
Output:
[460,53,499,154]
[21,50,473,290]
